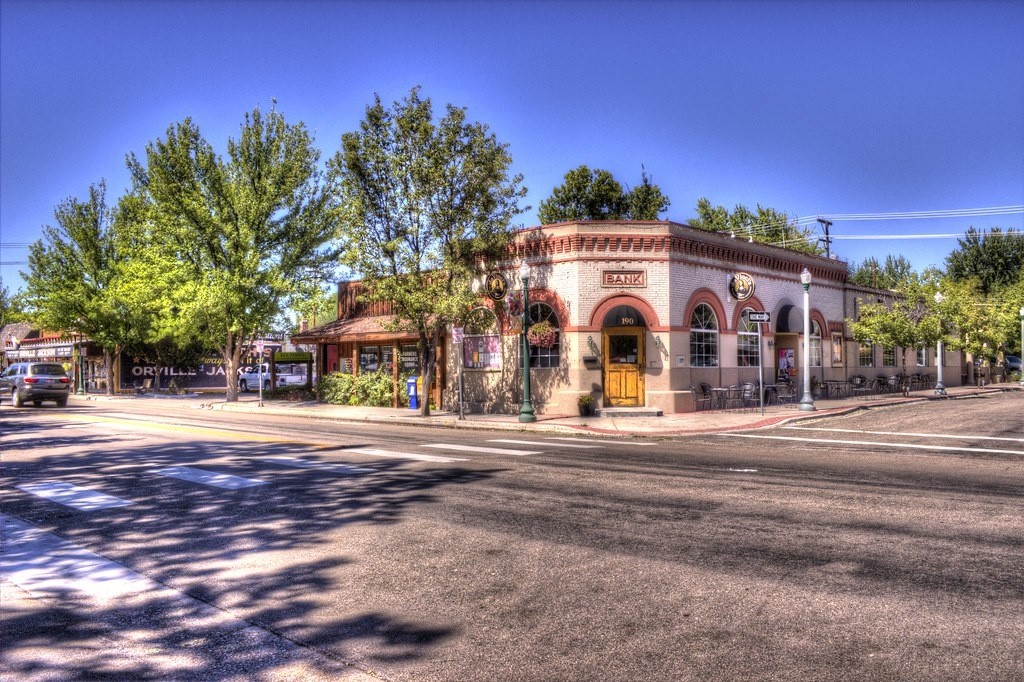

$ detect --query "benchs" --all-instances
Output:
[134,378,153,395]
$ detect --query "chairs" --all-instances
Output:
[37,369,46,373]
[688,378,797,415]
[818,383,828,400]
[848,372,933,401]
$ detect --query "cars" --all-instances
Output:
[238,362,307,395]
[989,355,1022,376]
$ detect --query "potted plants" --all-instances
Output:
[577,395,596,416]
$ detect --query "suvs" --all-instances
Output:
[0,361,70,408]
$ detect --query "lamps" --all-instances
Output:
[767,340,780,351]
[878,299,884,303]
[737,236,754,243]
[718,231,735,238]
[587,336,593,347]
[654,335,661,348]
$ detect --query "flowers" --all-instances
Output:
[526,320,558,349]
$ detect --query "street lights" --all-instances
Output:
[1018,307,1024,386]
[517,260,537,423]
[934,291,948,395]
[799,267,817,411]
[74,317,86,395]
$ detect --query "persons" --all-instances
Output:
[739,355,749,366]
[974,354,985,390]
[966,361,972,384]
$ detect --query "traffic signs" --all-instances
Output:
[748,311,772,323]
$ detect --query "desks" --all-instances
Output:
[709,387,745,414]
[823,380,851,400]
[764,384,787,409]
